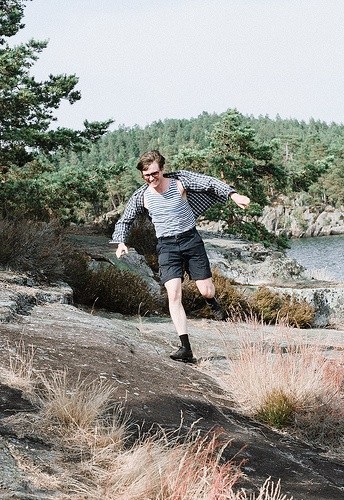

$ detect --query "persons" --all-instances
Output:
[112,150,250,360]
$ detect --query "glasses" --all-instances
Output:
[144,171,160,180]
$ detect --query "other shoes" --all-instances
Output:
[205,301,226,322]
[169,345,194,361]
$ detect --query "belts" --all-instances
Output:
[158,228,197,240]
